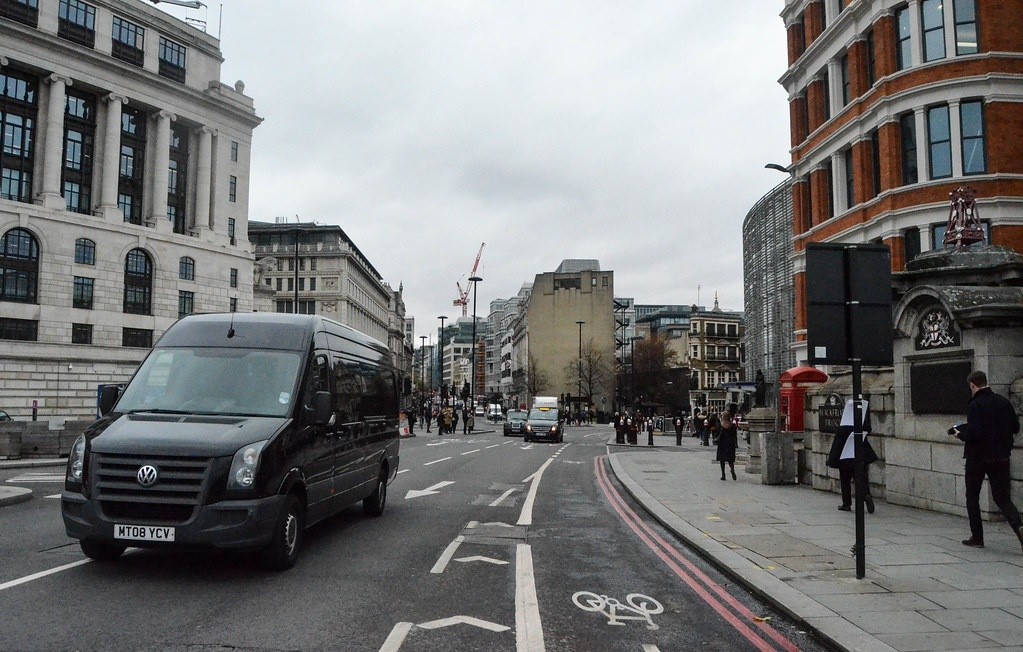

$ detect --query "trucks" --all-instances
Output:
[487,404,502,420]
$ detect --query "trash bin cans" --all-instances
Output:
[759,432,797,485]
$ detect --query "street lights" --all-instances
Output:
[419,335,427,407]
[468,277,483,430]
[437,316,448,409]
[429,346,435,400]
[288,227,305,313]
[576,320,585,425]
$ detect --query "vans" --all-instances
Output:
[523,409,564,442]
[61,311,399,571]
[503,409,529,437]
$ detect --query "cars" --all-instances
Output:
[475,408,484,416]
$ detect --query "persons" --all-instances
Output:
[613,409,642,444]
[953,370,1023,549]
[826,397,878,514]
[565,409,594,425]
[712,410,737,480]
[403,402,475,435]
[673,409,721,445]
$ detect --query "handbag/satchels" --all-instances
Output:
[863,440,878,465]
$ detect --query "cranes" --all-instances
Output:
[453,243,485,316]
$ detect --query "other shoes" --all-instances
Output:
[864,495,875,513]
[962,535,984,548]
[731,469,736,480]
[721,473,725,480]
[1016,525,1023,552]
[838,505,851,511]
